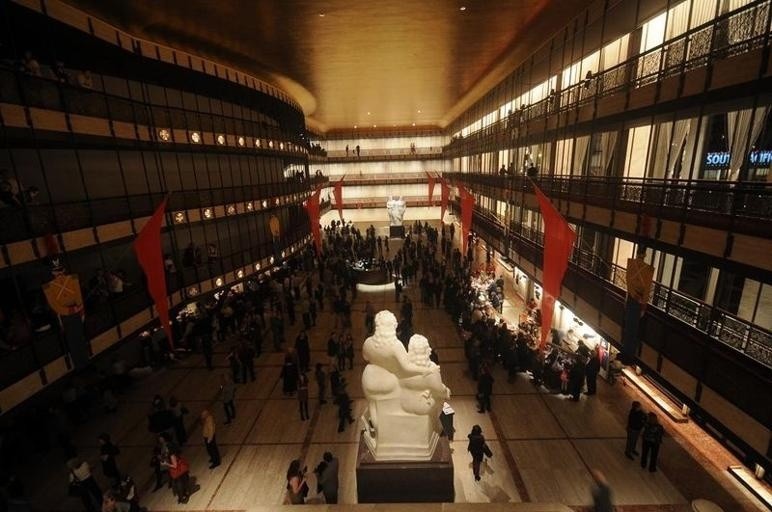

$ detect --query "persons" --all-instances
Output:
[466,425,493,482]
[312,143,320,150]
[316,170,322,176]
[589,469,614,512]
[527,162,537,176]
[356,145,360,156]
[0,220,389,512]
[296,170,305,182]
[624,401,664,473]
[500,163,512,175]
[346,145,348,157]
[386,220,600,413]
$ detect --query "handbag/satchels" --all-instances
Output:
[67,472,81,497]
[169,456,190,478]
